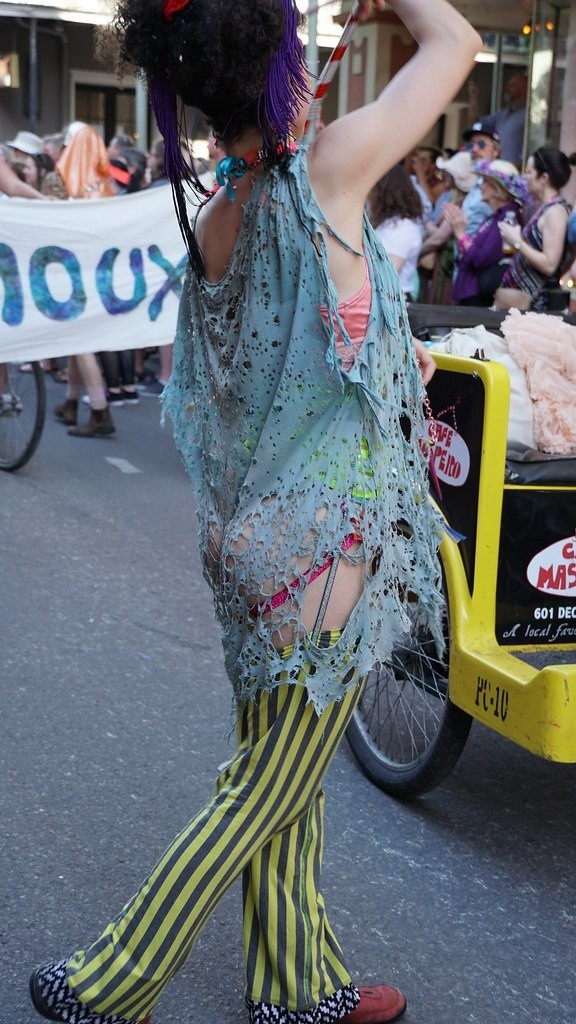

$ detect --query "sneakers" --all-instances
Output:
[124,388,141,404]
[81,389,125,407]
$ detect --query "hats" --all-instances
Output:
[5,131,43,155]
[470,158,524,198]
[436,152,478,193]
[463,121,501,143]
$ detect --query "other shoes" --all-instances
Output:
[30,964,152,1024]
[54,369,72,382]
[136,381,165,397]
[19,362,55,373]
[333,984,406,1024]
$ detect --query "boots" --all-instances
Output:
[68,409,115,436]
[53,399,78,425]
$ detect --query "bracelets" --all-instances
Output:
[458,233,473,252]
[513,241,522,250]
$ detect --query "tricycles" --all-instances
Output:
[340,296,576,802]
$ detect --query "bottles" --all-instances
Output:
[501,211,520,254]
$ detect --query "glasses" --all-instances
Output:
[467,140,495,151]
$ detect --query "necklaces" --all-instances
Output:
[212,140,297,204]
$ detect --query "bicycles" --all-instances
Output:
[1,361,46,473]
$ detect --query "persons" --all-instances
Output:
[28,1,484,1023]
[0,120,230,438]
[363,121,576,319]
[478,68,526,168]
